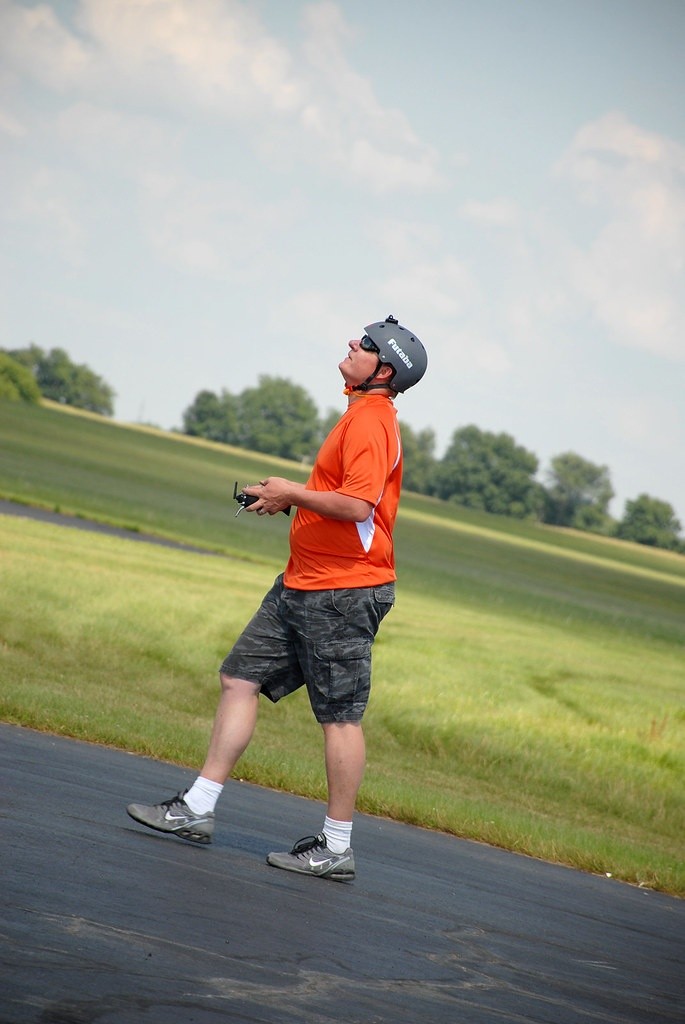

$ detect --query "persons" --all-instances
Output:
[127,315,427,880]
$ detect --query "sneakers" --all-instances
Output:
[126,788,217,844]
[266,831,356,880]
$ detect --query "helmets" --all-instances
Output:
[364,315,428,393]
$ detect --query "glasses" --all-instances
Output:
[359,334,380,354]
